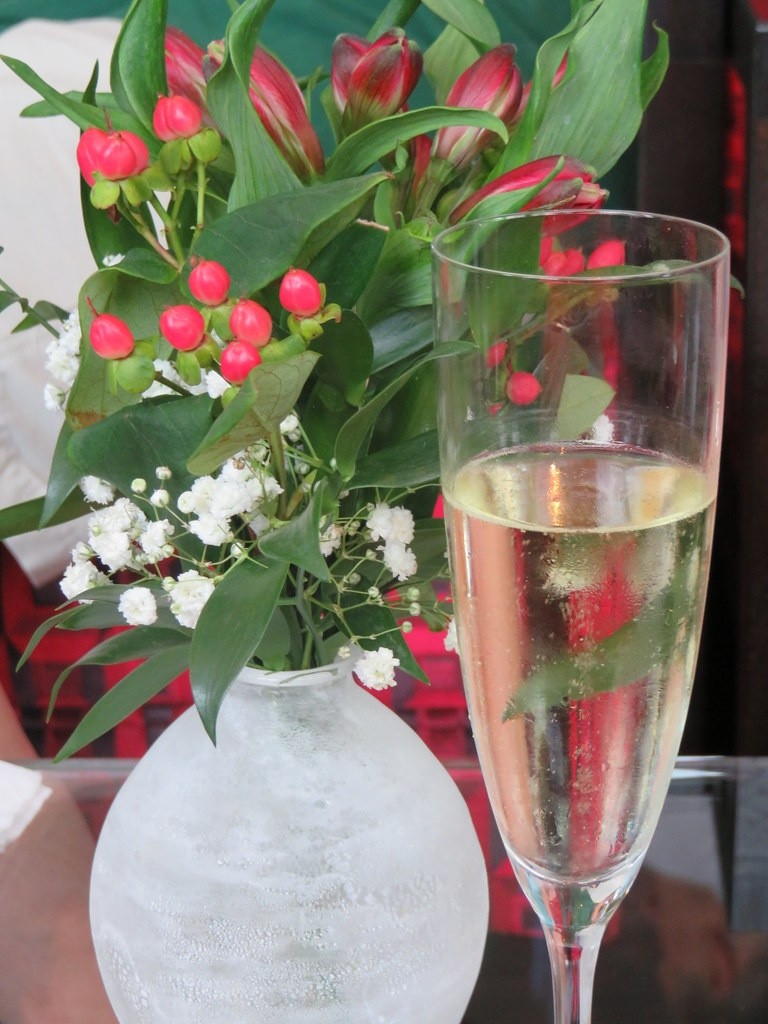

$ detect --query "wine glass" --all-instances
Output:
[428,208,732,1024]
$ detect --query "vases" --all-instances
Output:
[85,642,493,1024]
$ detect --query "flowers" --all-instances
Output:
[0,0,745,774]
